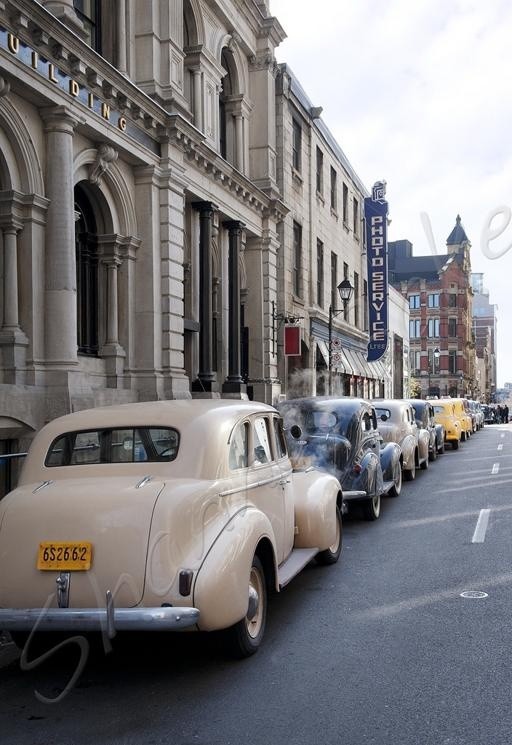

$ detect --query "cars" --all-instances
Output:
[275,396,512,521]
[2,399,346,656]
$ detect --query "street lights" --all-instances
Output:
[426,347,440,395]
[326,275,356,395]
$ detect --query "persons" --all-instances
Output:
[493,403,510,424]
